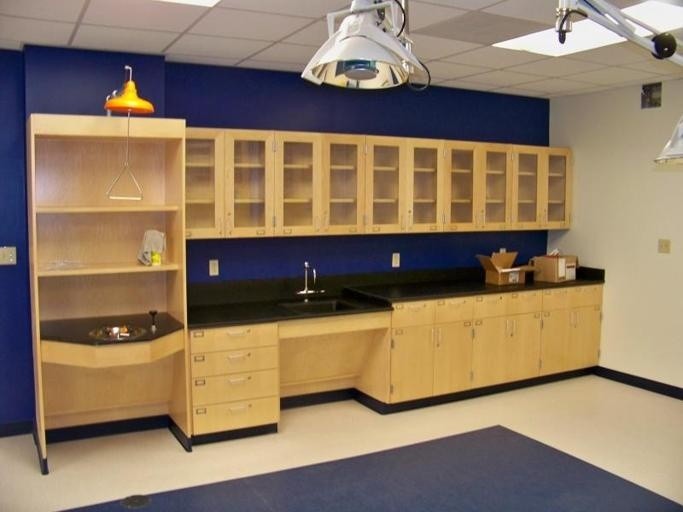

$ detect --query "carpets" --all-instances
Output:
[63,416,680,510]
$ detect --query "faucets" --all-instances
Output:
[297,260,318,294]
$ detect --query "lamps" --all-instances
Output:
[299,0,430,93]
[105,61,154,116]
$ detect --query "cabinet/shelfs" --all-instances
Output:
[470,288,540,395]
[442,138,513,234]
[19,107,194,478]
[363,132,443,235]
[176,119,276,243]
[272,127,365,239]
[538,284,603,381]
[509,144,571,232]
[184,318,280,446]
[352,297,472,415]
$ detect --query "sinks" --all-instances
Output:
[272,294,367,313]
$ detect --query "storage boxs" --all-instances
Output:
[528,253,578,283]
[475,247,540,286]
